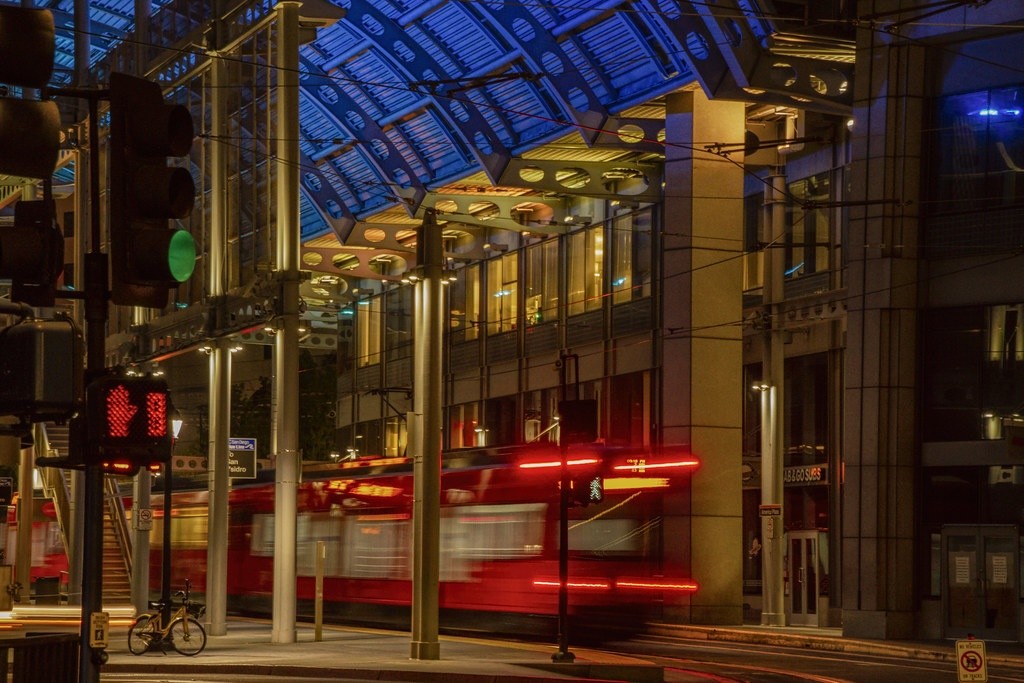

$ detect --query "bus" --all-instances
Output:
[226,430,669,647]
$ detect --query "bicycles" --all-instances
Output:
[127,578,206,657]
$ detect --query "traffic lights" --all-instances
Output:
[86,364,171,464]
[107,72,200,306]
[0,0,63,310]
